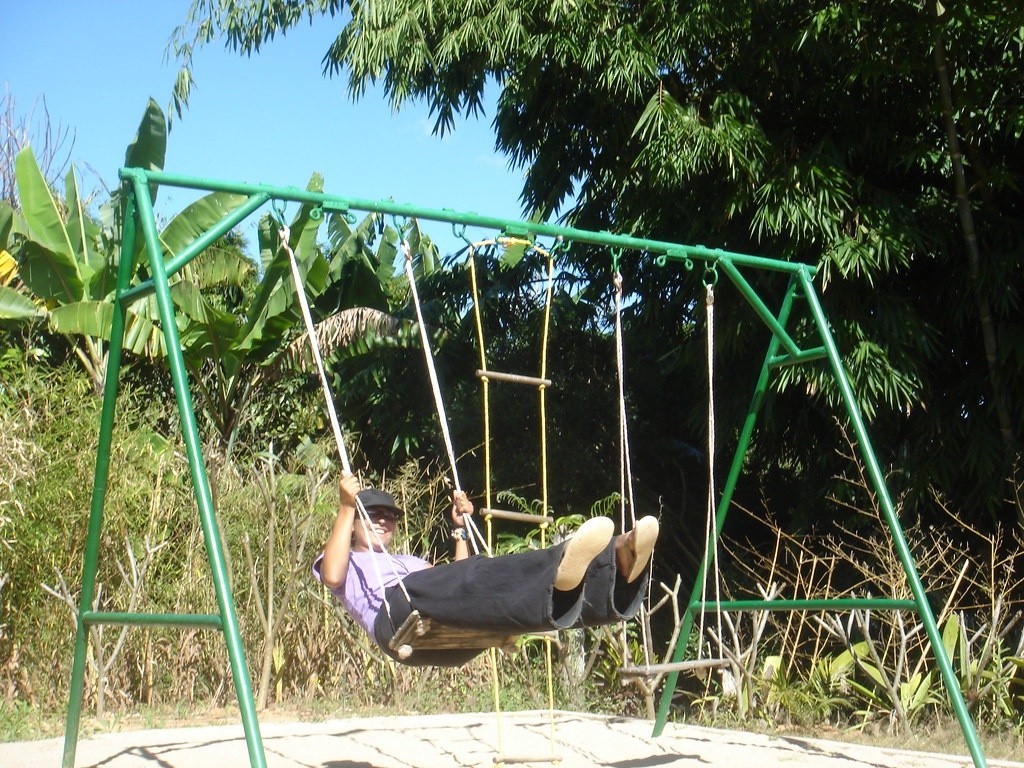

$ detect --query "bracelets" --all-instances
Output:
[451,525,468,541]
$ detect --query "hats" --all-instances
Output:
[355,488,405,520]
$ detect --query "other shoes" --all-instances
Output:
[625,515,659,584]
[554,516,616,591]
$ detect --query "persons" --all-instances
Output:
[313,469,661,667]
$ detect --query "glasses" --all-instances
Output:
[358,508,399,522]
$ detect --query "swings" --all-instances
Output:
[276,226,523,658]
[612,270,734,678]
